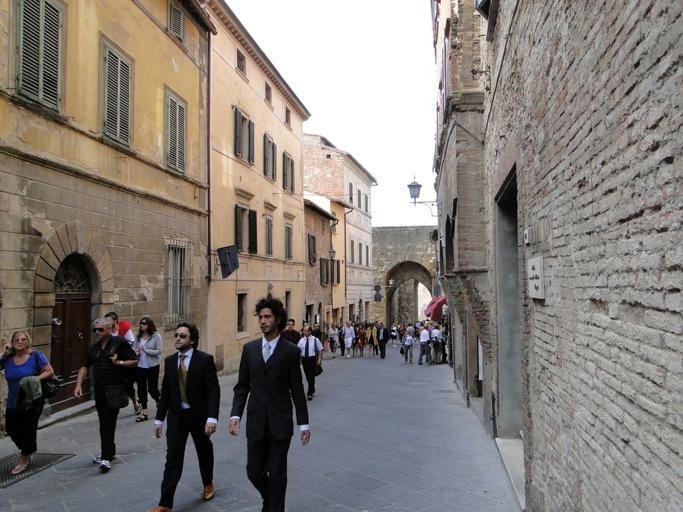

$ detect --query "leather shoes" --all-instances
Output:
[307,395,312,400]
[203,484,213,500]
[150,506,172,512]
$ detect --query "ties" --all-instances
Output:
[305,338,308,358]
[263,343,270,364]
[178,354,187,404]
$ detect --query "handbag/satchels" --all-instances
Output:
[313,360,322,376]
[34,350,60,398]
[399,347,404,357]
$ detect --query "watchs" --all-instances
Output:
[119,360,125,366]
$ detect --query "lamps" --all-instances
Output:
[407,175,441,217]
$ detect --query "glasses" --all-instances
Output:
[174,333,186,338]
[139,322,147,325]
[91,328,104,332]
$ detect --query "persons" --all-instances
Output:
[226,297,311,511]
[71,317,139,472]
[148,322,221,511]
[282,319,300,344]
[103,312,140,415]
[296,325,323,401]
[299,319,447,364]
[0,330,53,473]
[133,317,161,422]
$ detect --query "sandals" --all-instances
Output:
[135,414,148,422]
[134,400,140,415]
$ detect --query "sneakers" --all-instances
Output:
[98,460,111,470]
[11,463,28,476]
[93,455,101,463]
